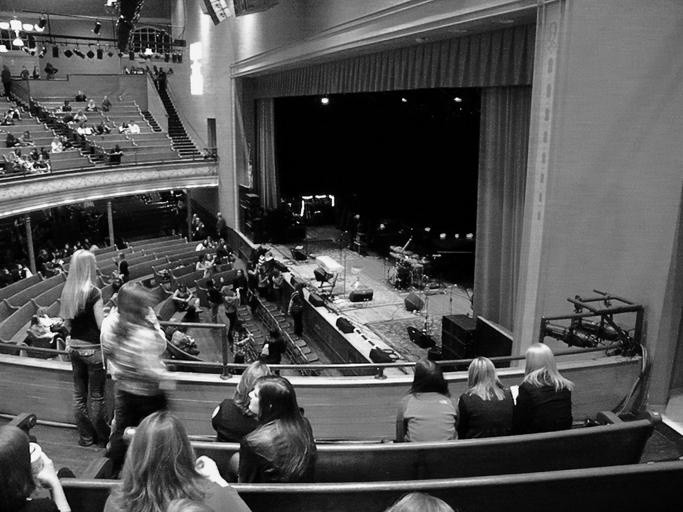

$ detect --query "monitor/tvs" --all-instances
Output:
[476,315,514,369]
[239,184,251,211]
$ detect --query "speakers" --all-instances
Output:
[428,346,442,360]
[97,49,103,59]
[204,0,232,26]
[314,267,333,282]
[53,47,58,57]
[441,314,477,372]
[404,292,424,312]
[407,326,436,349]
[349,289,373,302]
[129,52,134,60]
[289,247,307,260]
[245,194,263,244]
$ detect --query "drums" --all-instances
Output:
[388,265,399,285]
[410,264,425,276]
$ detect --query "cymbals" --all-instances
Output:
[390,245,407,254]
[388,251,398,258]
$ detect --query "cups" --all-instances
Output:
[30,439,45,476]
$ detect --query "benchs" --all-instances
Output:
[1,96,179,182]
[0,232,683,512]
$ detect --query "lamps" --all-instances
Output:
[22,35,183,62]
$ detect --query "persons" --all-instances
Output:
[455,357,515,439]
[202,147,216,162]
[380,358,458,445]
[2,188,318,511]
[513,343,574,436]
[0,62,174,176]
[383,490,456,511]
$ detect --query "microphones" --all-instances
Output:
[433,254,442,257]
